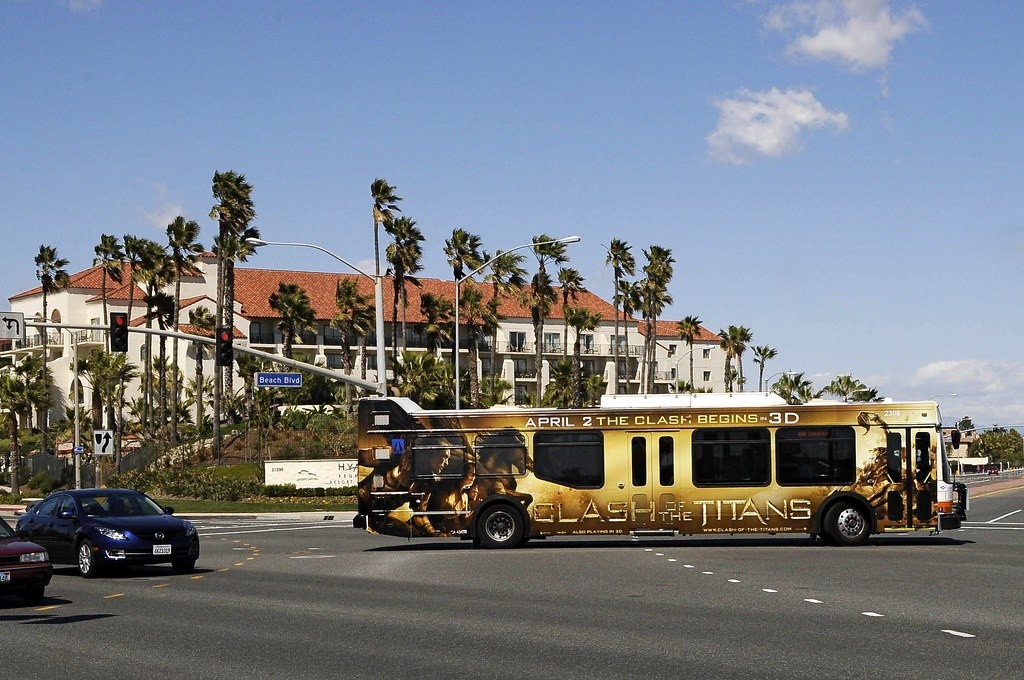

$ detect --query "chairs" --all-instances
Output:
[510,344,530,352]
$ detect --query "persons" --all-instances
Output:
[357,433,480,537]
[83,499,98,515]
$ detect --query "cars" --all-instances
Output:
[988,466,999,476]
[0,515,54,606]
[15,488,200,579]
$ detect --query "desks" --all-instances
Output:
[520,347,525,352]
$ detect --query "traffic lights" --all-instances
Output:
[110,312,129,352]
[216,327,234,366]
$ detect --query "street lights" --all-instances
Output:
[245,237,387,398]
[32,315,81,491]
[676,346,716,393]
[456,236,581,410]
[766,372,797,393]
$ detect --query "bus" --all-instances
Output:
[352,390,970,550]
